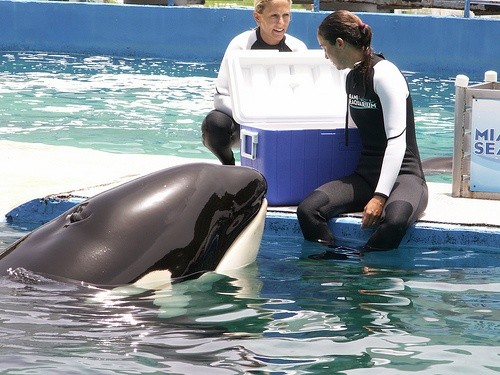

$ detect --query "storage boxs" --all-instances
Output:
[225,44,364,208]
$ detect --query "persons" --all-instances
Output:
[201,1,312,168]
[287,10,428,256]
